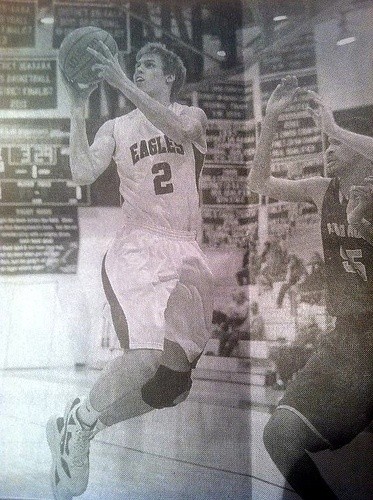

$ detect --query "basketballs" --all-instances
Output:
[59,25,119,85]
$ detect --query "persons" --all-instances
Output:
[211,237,334,392]
[245,73,373,500]
[346,177,373,245]
[45,41,219,500]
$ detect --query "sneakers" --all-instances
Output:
[45,415,89,500]
[56,398,97,496]
